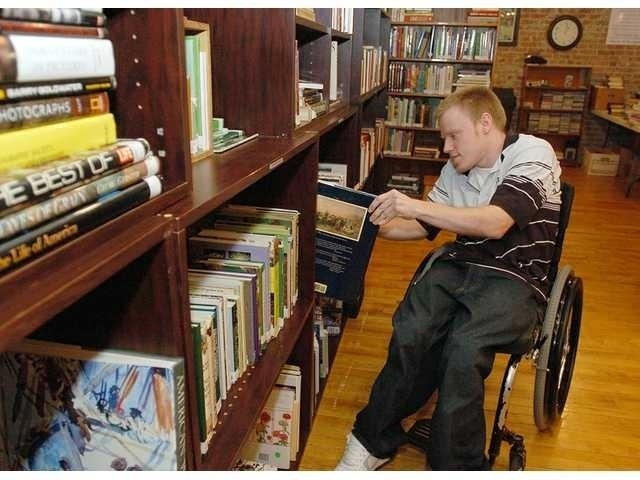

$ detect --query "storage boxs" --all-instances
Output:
[581,84,629,175]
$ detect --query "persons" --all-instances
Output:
[331,85,564,471]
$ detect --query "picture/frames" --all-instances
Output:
[608,9,640,47]
[498,7,520,48]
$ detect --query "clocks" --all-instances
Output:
[548,14,583,52]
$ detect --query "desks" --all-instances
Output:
[589,103,639,198]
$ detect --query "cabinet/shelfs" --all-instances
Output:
[2,7,396,471]
[385,20,496,175]
[516,57,592,167]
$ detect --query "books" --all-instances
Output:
[1,339,186,471]
[1,7,165,276]
[515,74,640,140]
[296,8,389,337]
[186,202,300,470]
[383,169,424,200]
[389,8,500,160]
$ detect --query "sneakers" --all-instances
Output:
[334,430,392,470]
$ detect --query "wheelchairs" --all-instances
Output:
[403,182,585,470]
